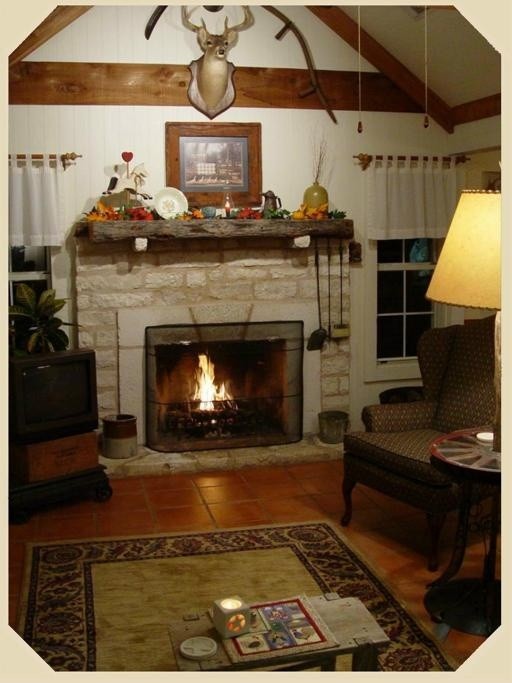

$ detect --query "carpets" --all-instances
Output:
[15,520,460,672]
[424,577,501,636]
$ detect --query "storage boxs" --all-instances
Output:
[14,431,98,484]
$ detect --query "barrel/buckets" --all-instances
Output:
[318,410,350,445]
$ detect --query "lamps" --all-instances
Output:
[424,179,501,452]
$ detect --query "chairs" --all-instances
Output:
[343,314,496,572]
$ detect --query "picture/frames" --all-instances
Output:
[165,122,262,207]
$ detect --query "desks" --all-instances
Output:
[430,426,501,619]
[9,465,112,525]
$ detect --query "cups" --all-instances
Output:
[201,207,216,219]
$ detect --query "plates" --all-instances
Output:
[154,187,188,219]
[476,432,493,442]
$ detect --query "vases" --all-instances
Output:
[304,183,328,213]
